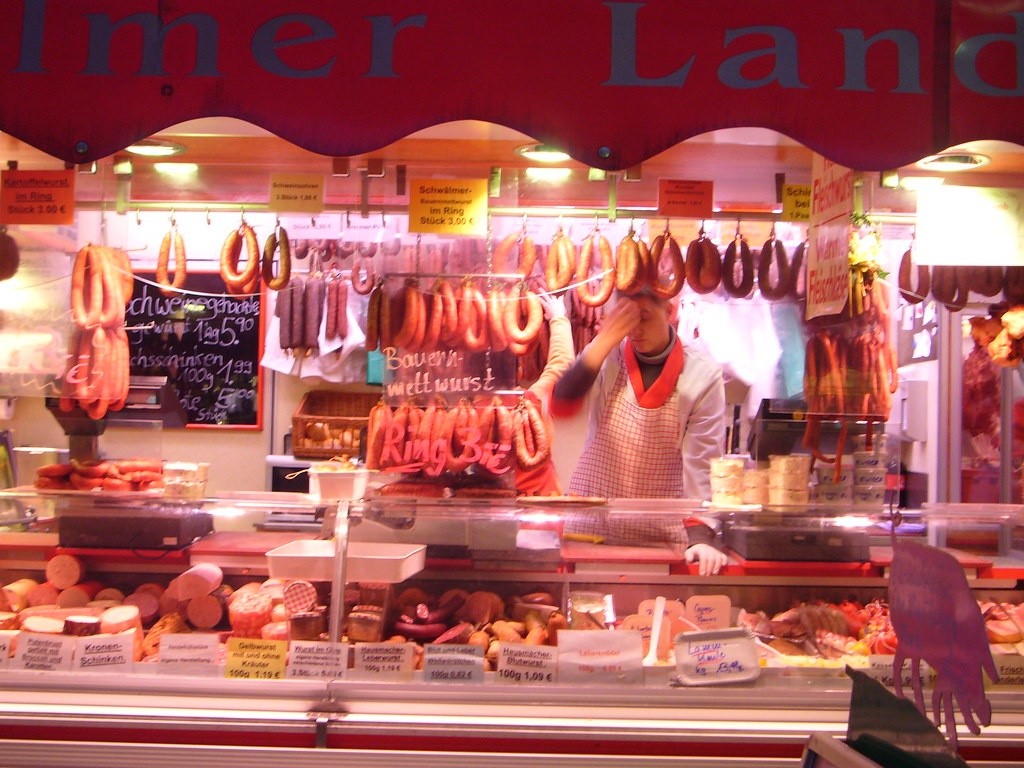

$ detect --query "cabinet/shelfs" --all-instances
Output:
[0,478,1024,768]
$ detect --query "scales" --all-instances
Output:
[58,506,215,549]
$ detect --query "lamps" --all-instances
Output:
[124,142,185,157]
[914,153,992,173]
[901,177,946,192]
[513,143,571,163]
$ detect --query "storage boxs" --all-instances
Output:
[291,390,382,459]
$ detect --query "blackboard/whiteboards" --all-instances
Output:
[113,268,269,433]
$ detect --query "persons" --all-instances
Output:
[469,289,576,572]
[553,282,729,583]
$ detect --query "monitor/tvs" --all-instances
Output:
[46,376,188,429]
[748,399,884,461]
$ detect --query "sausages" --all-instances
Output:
[155,232,188,295]
[366,401,552,477]
[900,249,1024,315]
[56,246,133,421]
[220,224,810,398]
[34,459,163,496]
[387,593,566,674]
[783,606,850,640]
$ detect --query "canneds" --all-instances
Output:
[284,581,385,643]
